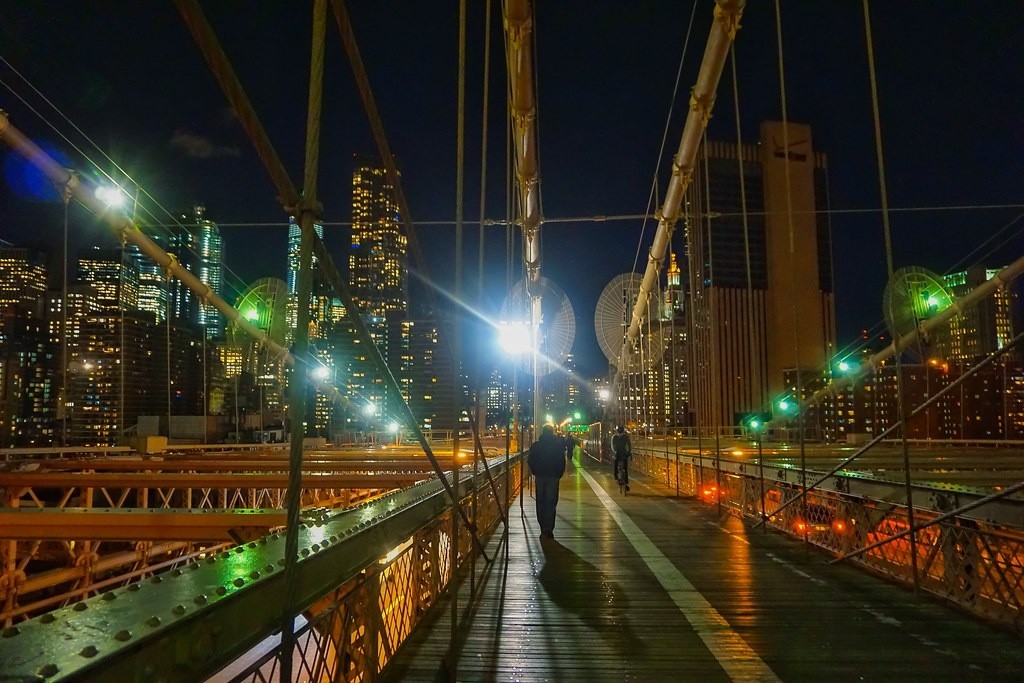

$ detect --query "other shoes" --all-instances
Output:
[540,533,554,538]
[614,475,618,480]
[626,485,630,491]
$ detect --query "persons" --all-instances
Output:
[528,424,565,537]
[559,433,575,459]
[611,426,632,492]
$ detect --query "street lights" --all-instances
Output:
[497,323,531,442]
